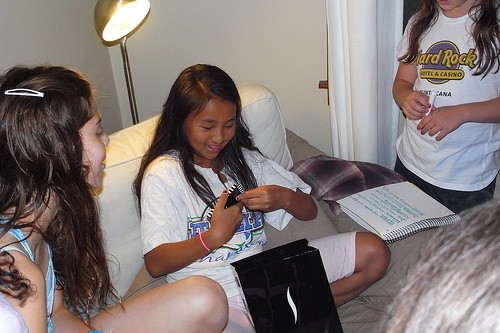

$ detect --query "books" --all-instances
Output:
[335,181,460,244]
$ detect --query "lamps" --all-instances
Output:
[94,0,151,125]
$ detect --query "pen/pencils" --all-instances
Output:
[426,92,437,116]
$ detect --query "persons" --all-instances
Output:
[0,64,228,333]
[390,0,500,214]
[380,197,500,332]
[131,65,391,333]
[0,251,38,333]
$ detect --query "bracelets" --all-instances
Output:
[199,232,211,251]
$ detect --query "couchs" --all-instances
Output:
[66,80,436,333]
